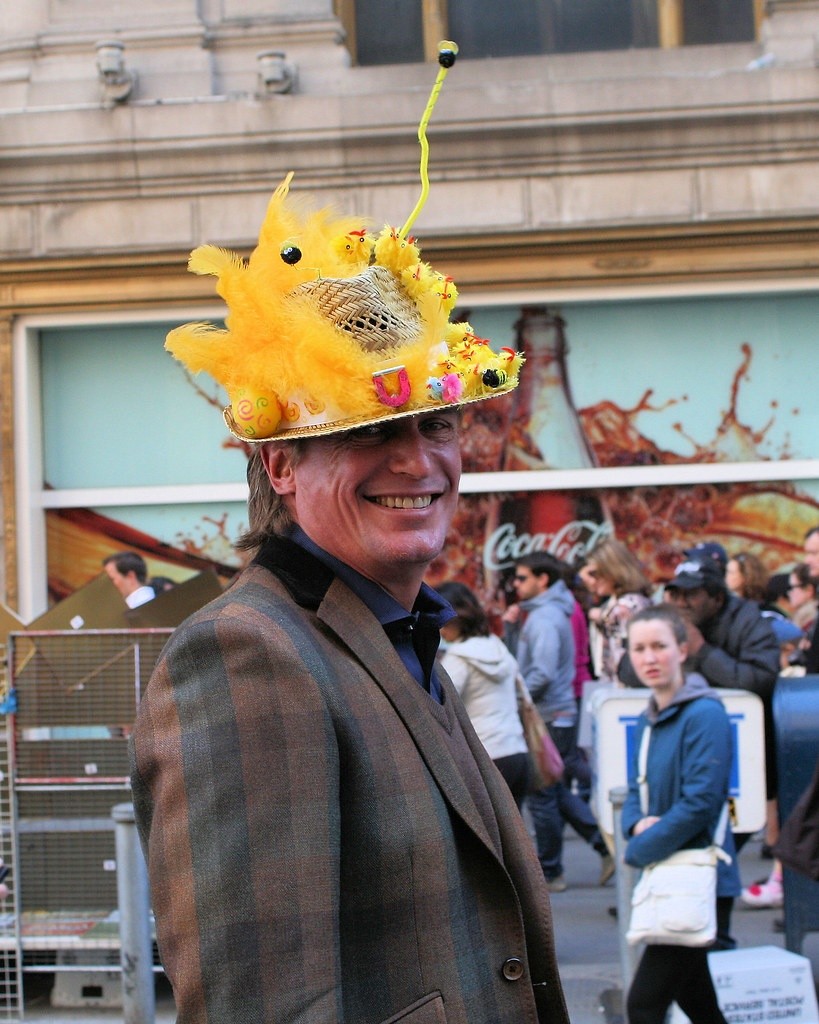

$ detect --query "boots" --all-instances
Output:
[715,898,739,948]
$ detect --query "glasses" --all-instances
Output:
[515,575,536,581]
[787,584,805,592]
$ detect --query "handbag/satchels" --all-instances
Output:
[624,846,731,949]
[515,675,562,790]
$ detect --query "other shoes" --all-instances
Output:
[740,878,784,907]
[547,877,567,892]
[598,855,616,884]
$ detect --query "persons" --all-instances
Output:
[432,527,819,1024]
[103,551,175,997]
[130,173,571,1024]
[0,951,56,1005]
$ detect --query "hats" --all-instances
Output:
[663,558,724,593]
[683,543,728,569]
[164,42,520,444]
